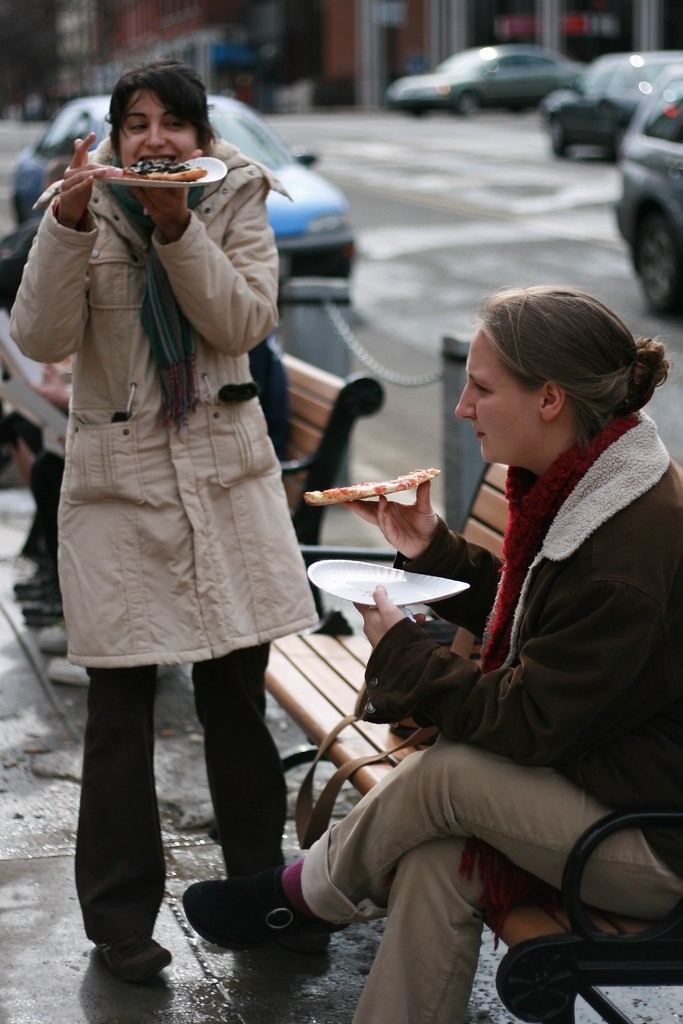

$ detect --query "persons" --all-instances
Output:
[13,335,292,687]
[179,286,683,1023]
[6,60,321,975]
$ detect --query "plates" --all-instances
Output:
[306,560,471,605]
[96,157,228,188]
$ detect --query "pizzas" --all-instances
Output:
[122,156,208,181]
[303,468,441,506]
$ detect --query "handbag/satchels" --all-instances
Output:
[295,612,476,848]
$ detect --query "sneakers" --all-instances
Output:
[95,934,171,983]
[269,929,329,949]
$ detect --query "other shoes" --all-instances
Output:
[21,593,68,624]
[39,623,69,653]
[46,658,90,686]
[13,565,60,597]
[184,864,351,949]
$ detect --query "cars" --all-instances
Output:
[381,44,589,116]
[540,51,683,156]
[611,61,683,316]
[9,92,357,298]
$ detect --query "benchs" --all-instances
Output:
[263,461,683,1024]
[278,351,388,621]
